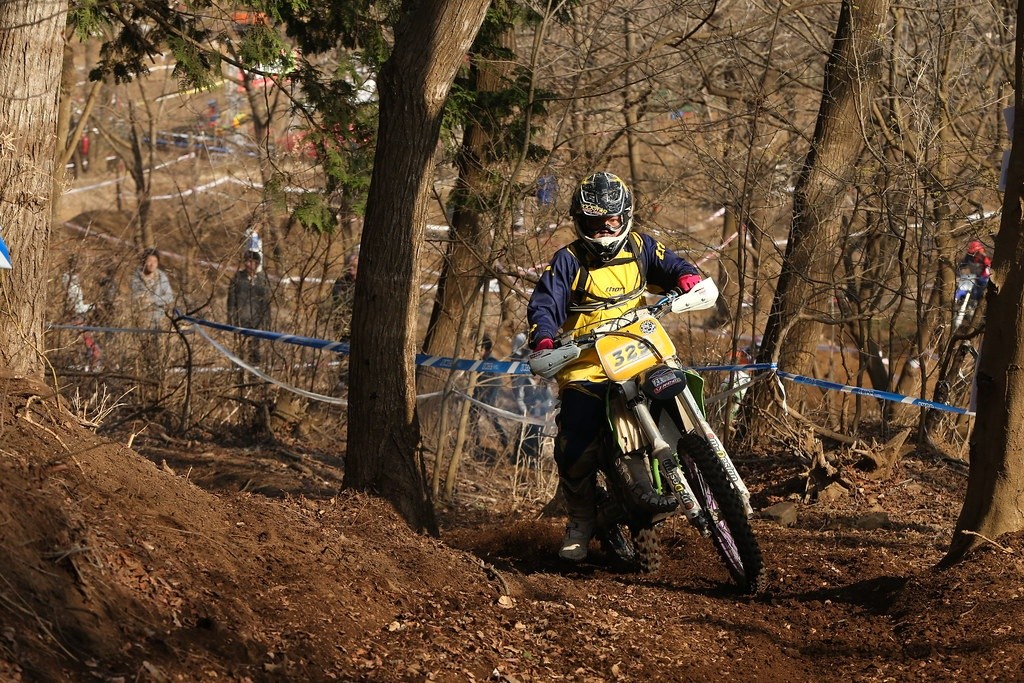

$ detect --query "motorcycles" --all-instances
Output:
[527,276,768,601]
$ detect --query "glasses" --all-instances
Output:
[585,215,625,235]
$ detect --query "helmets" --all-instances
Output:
[569,172,635,263]
[967,242,984,263]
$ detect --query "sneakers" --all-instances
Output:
[560,520,594,560]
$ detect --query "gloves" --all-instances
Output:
[533,337,554,352]
[677,275,701,293]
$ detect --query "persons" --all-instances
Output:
[131,249,174,383]
[526,171,703,561]
[59,253,117,384]
[226,250,270,370]
[333,253,359,396]
[244,215,262,274]
[955,240,990,307]
[470,332,554,472]
